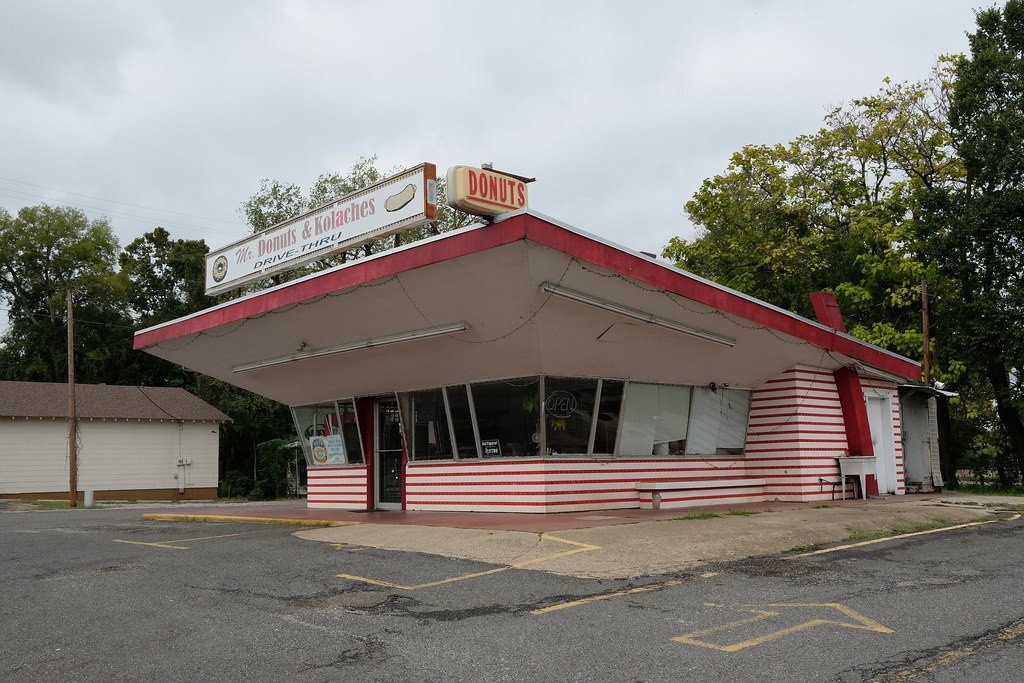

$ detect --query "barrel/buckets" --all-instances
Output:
[654,442,670,455]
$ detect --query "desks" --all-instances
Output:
[838,456,878,501]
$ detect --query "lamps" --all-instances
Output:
[539,282,737,348]
[231,322,471,374]
[933,381,945,390]
